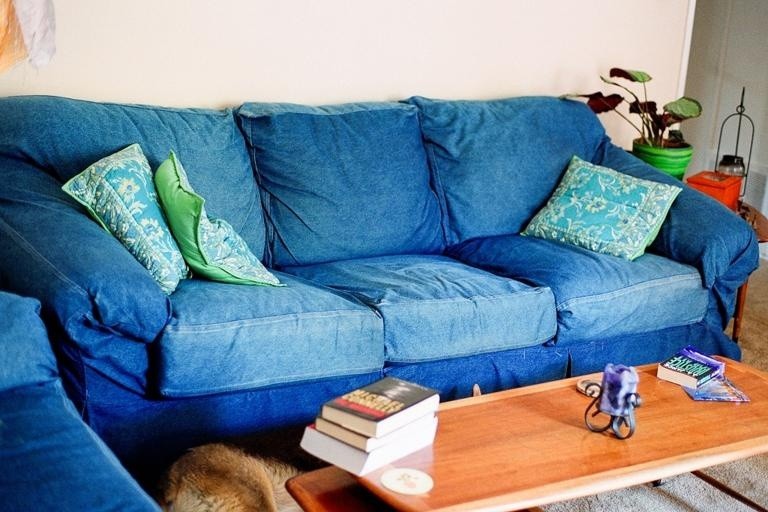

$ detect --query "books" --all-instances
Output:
[298,375,441,477]
[657,344,726,391]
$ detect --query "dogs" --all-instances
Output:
[164,443,305,512]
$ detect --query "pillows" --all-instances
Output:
[520,153,681,263]
[59,139,283,295]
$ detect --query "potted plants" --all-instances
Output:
[559,68,745,213]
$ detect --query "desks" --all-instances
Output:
[718,200,768,343]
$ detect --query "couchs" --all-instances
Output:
[0,94,759,483]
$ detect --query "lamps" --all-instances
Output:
[715,87,755,210]
